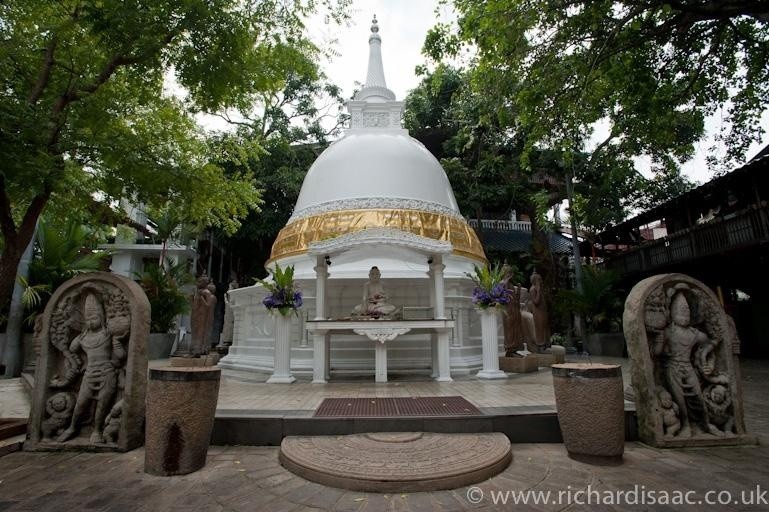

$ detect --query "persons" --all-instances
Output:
[353,265,396,315]
[40,293,131,443]
[652,292,736,440]
[498,258,549,358]
[186,269,239,358]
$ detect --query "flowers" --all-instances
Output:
[252,259,304,319]
[462,258,516,312]
